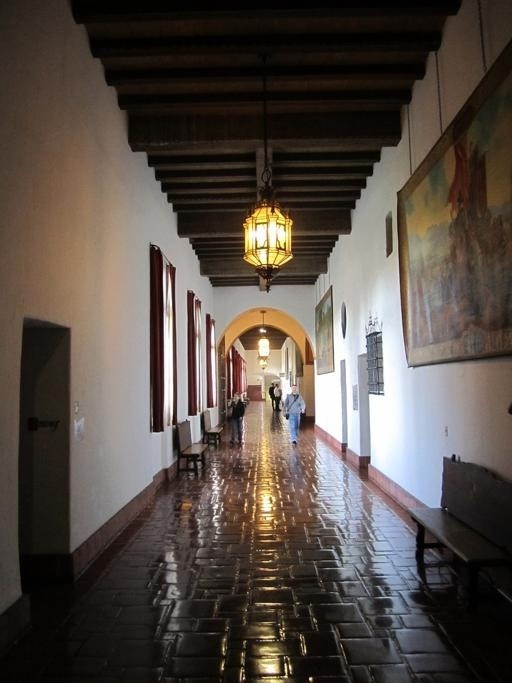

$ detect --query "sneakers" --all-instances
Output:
[229,439,243,446]
[292,436,299,445]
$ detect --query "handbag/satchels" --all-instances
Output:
[285,412,290,420]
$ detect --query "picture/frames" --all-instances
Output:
[187,289,203,415]
[150,245,177,433]
[397,42,511,369]
[315,285,334,375]
[305,338,314,365]
[206,313,219,408]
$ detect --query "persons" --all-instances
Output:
[226,392,245,448]
[269,383,275,411]
[274,384,281,411]
[282,385,306,445]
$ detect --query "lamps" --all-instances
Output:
[258,310,269,362]
[242,40,293,297]
[259,359,267,370]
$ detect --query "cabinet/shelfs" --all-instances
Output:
[248,385,262,401]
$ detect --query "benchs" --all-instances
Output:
[203,406,224,450]
[240,392,250,408]
[407,456,512,625]
[176,419,208,481]
